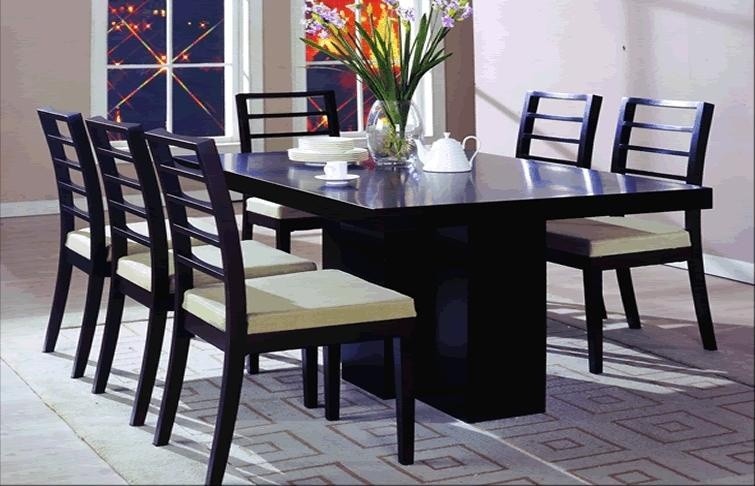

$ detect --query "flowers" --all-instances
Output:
[298,0,476,163]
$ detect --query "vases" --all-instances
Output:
[366,97,424,166]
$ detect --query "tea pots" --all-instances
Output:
[414,132,479,173]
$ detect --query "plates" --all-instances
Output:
[314,174,360,185]
[287,138,369,167]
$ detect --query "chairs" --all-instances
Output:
[547,96,718,376]
[237,90,341,252]
[85,115,319,427]
[514,89,603,173]
[36,107,220,378]
[144,126,423,485]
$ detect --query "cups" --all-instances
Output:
[323,161,347,178]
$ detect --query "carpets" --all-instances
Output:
[2,304,754,484]
